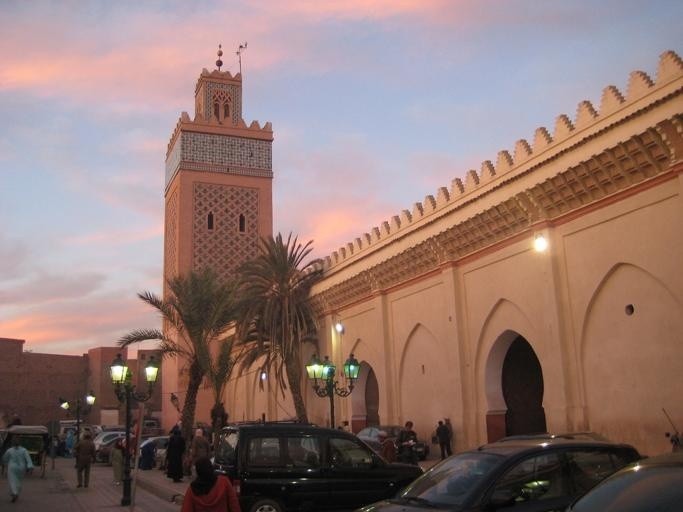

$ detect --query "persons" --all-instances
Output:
[435,419,452,460]
[664,430,682,453]
[1,437,34,501]
[181,458,241,511]
[72,430,97,489]
[393,420,418,466]
[444,417,452,439]
[5,411,210,482]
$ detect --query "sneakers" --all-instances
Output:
[84,485,89,488]
[10,494,18,502]
[173,479,183,483]
[76,485,82,488]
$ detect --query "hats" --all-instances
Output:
[195,457,213,472]
[196,428,203,437]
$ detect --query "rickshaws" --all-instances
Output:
[0,423,51,478]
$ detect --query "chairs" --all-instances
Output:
[255,446,278,463]
[292,448,307,461]
[302,451,317,468]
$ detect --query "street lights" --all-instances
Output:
[105,352,160,507]
[302,352,362,429]
[52,389,96,468]
